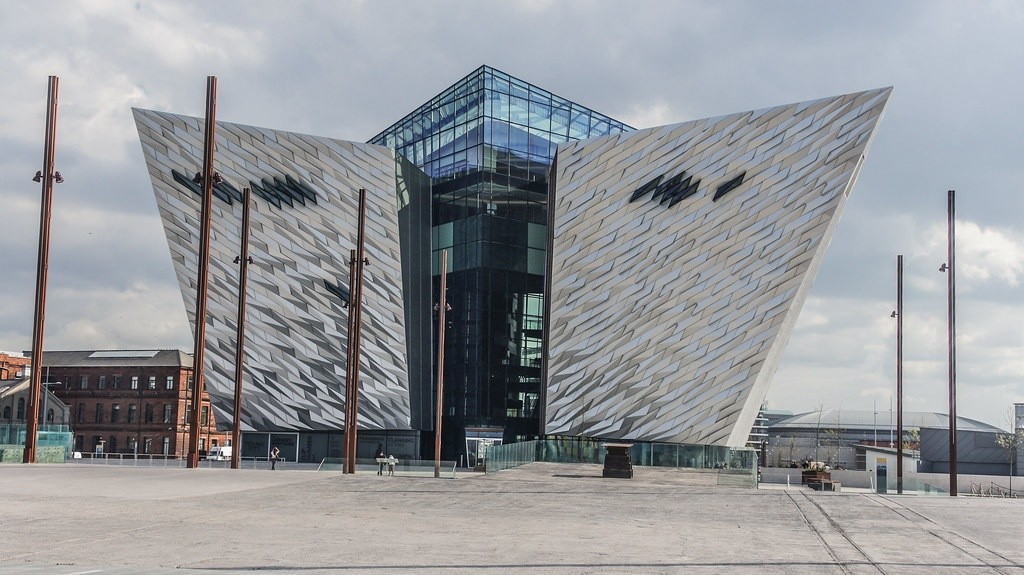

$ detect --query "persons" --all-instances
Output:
[790,461,798,468]
[376,451,386,476]
[387,455,395,477]
[715,462,727,469]
[270,447,280,470]
[802,461,810,469]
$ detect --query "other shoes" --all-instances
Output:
[376,471,379,475]
[391,474,394,476]
[380,473,382,476]
[272,468,275,470]
[388,474,391,476]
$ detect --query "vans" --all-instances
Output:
[780,459,796,468]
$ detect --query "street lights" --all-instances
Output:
[348,257,371,475]
[186,171,223,468]
[343,299,355,472]
[231,255,256,469]
[434,302,452,476]
[21,170,65,464]
[41,382,63,431]
[938,262,959,496]
[891,310,903,494]
[874,412,880,447]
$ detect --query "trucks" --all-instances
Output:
[207,447,232,462]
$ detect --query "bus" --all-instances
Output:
[20,431,73,459]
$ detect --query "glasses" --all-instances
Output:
[276,450,278,451]
